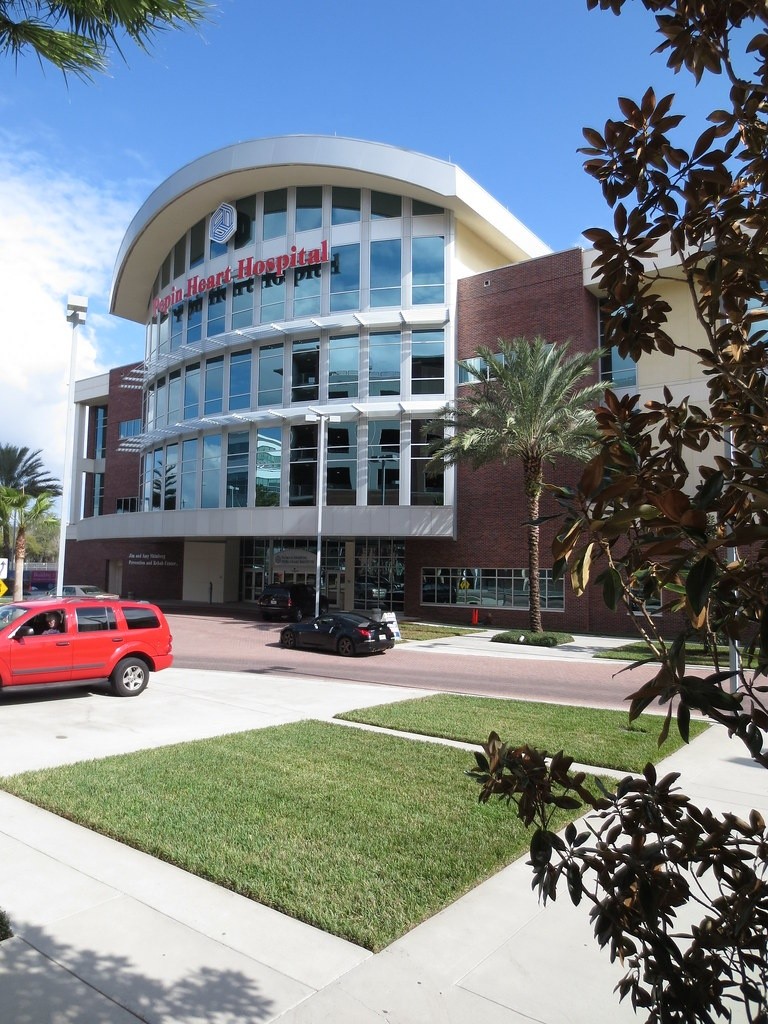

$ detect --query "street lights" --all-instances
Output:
[53,295,92,596]
[304,414,342,622]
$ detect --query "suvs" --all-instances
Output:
[256,582,329,622]
[0,596,174,697]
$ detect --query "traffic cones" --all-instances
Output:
[470,608,479,626]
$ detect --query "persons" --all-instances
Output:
[39,612,64,634]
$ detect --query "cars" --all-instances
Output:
[355,582,386,599]
[357,575,405,598]
[279,612,395,656]
[32,586,39,591]
[383,580,457,610]
[44,585,121,601]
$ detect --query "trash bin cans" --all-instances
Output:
[372,608,381,622]
